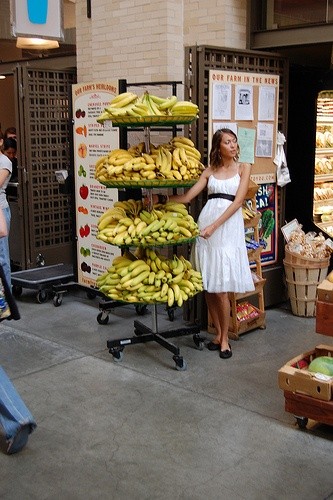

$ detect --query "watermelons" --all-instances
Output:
[308,356,333,377]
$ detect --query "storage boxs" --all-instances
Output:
[277,344,333,402]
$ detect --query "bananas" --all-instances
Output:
[97,199,200,246]
[96,248,204,307]
[95,136,205,182]
[96,90,200,123]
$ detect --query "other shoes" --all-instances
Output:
[220,343,232,359]
[1,309,11,319]
[207,341,221,351]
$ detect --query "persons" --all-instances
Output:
[0,128,18,320]
[146,128,252,359]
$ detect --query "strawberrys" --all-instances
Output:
[79,224,90,238]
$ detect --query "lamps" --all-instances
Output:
[15,34,59,50]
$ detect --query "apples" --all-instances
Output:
[80,184,89,199]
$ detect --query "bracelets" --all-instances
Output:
[157,193,168,204]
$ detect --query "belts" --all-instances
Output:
[208,193,236,202]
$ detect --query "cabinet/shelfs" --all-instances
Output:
[287,89,333,242]
[208,179,267,341]
[93,114,205,370]
